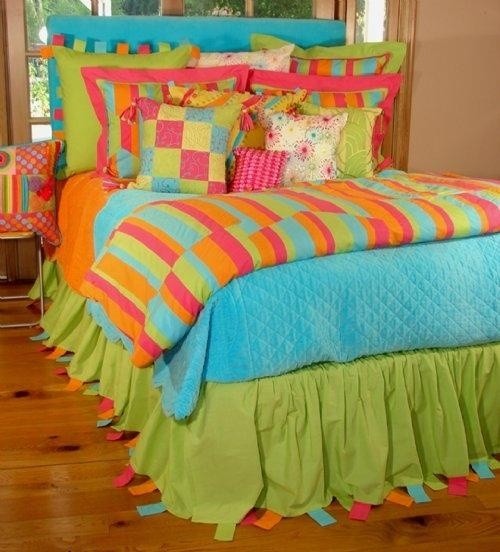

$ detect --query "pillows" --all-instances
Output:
[298,100,384,181]
[231,145,290,193]
[167,82,308,150]
[246,33,406,78]
[262,107,351,183]
[123,96,238,194]
[246,68,402,173]
[195,44,295,76]
[0,137,65,246]
[52,44,201,178]
[52,37,186,176]
[77,64,252,194]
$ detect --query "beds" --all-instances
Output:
[28,13,500,524]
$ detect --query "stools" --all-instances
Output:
[0,231,44,329]
